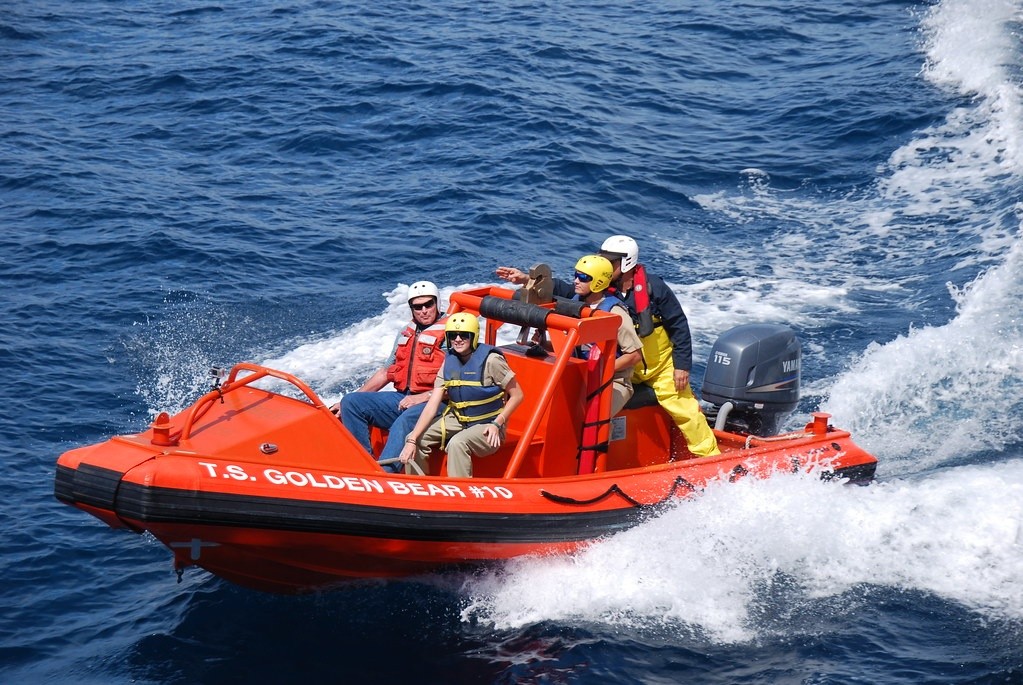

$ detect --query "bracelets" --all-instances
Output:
[490,421,501,429]
[499,414,505,423]
[406,439,417,445]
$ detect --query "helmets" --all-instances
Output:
[408,280,441,315]
[445,312,480,349]
[601,235,639,273]
[575,254,613,293]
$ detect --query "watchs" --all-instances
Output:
[428,390,433,396]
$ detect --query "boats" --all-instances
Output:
[54,283,878,600]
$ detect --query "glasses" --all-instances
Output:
[447,331,474,340]
[574,271,593,282]
[410,298,436,310]
[609,256,622,265]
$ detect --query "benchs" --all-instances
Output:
[367,423,544,478]
[608,405,670,471]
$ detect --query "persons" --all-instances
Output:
[496,235,723,457]
[399,313,524,479]
[330,279,449,474]
[571,254,645,444]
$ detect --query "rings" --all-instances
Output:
[405,404,407,405]
